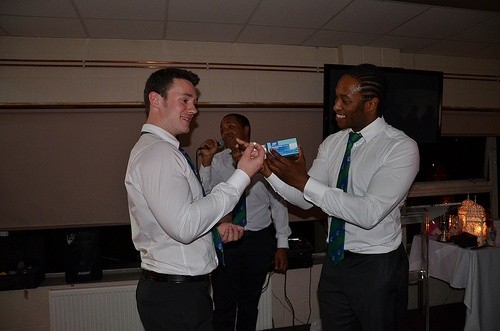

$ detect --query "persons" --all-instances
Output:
[124,67,266,331]
[232,63,420,331]
[198,113,292,331]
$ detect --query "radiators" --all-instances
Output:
[49,272,273,331]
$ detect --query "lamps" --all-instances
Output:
[458,191,488,242]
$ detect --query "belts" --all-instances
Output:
[344,242,405,258]
[141,268,214,284]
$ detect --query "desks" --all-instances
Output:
[408,220,500,331]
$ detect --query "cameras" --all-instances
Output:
[266,137,300,157]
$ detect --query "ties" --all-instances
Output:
[230,162,247,227]
[179,148,227,267]
[325,132,362,266]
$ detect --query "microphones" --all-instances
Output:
[198,139,224,151]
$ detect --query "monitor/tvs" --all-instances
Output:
[322,63,444,181]
